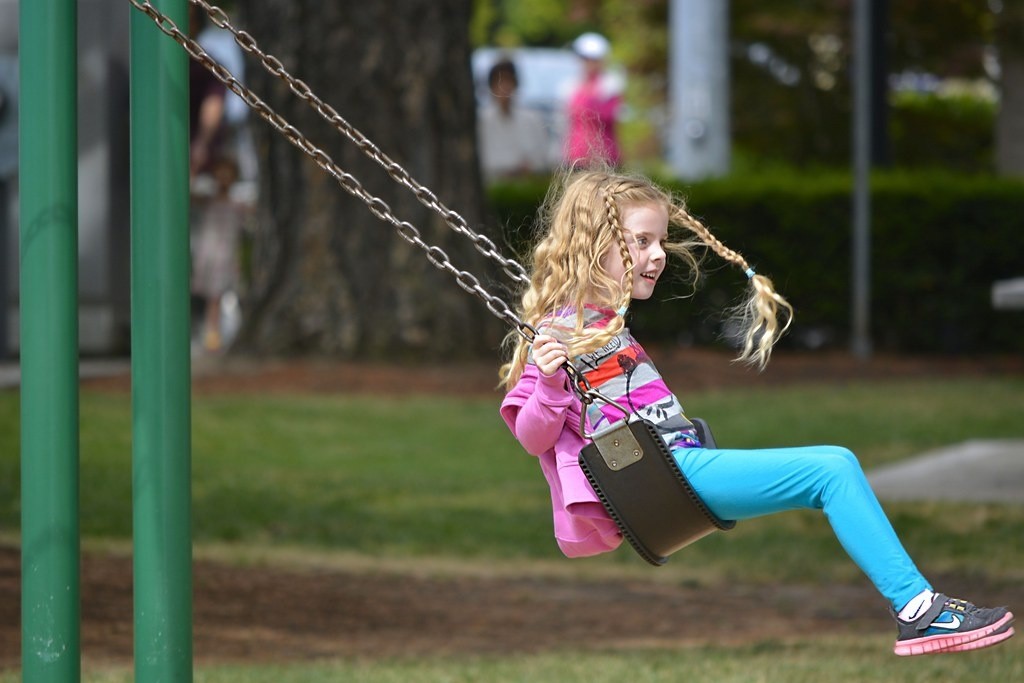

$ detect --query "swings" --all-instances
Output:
[129,0,736,566]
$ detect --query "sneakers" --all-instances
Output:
[894,592,1015,656]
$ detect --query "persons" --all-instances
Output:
[497,170,1017,659]
[189,0,263,351]
[476,29,630,194]
